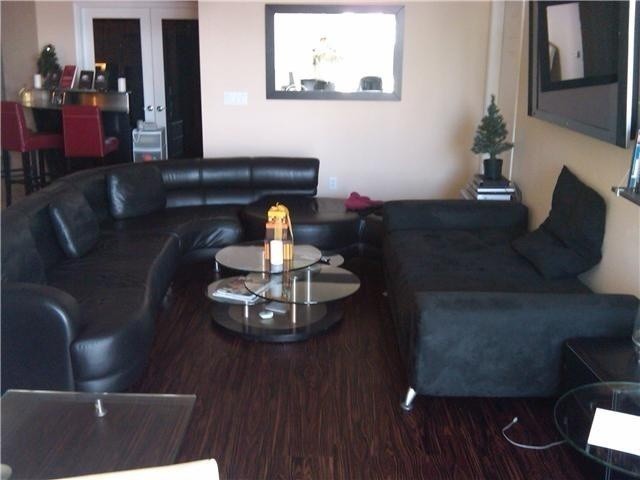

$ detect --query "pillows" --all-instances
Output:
[106,164,167,220]
[511,164,607,281]
[47,190,101,258]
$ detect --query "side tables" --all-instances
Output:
[559,336,640,480]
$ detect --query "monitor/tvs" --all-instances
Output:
[527,0,639,150]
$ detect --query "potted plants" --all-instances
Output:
[469,94,515,178]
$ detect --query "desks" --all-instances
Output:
[2,387,197,480]
[552,379,640,480]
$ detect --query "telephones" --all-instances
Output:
[132,119,157,143]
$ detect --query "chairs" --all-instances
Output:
[1,100,64,208]
[60,103,120,174]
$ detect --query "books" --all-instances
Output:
[212,276,270,303]
[467,178,515,201]
[627,129,640,195]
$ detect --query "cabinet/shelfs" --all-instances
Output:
[131,127,167,162]
[16,86,132,182]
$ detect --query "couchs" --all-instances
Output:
[1,156,320,395]
[373,199,640,412]
[239,195,361,256]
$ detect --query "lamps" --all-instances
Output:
[22,88,32,103]
